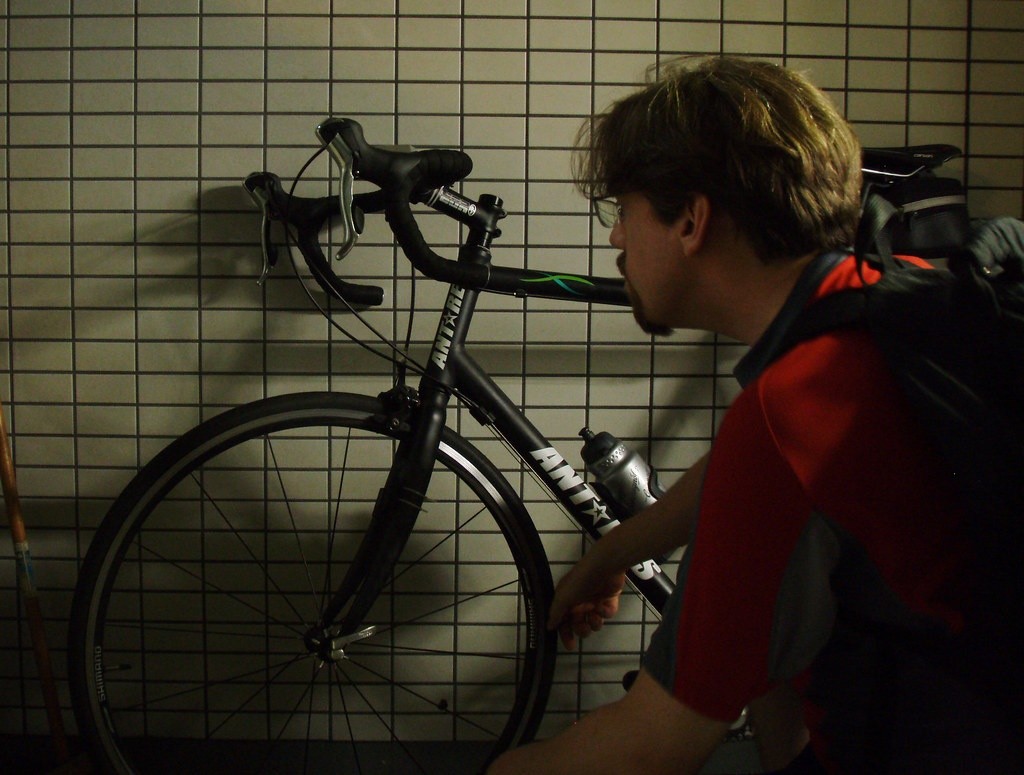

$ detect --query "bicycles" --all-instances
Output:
[62,115,966,774]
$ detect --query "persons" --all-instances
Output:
[488,57,1024,775]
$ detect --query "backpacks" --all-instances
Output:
[783,195,1024,725]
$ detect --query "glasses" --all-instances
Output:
[592,188,640,229]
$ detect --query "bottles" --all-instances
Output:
[576,425,679,562]
